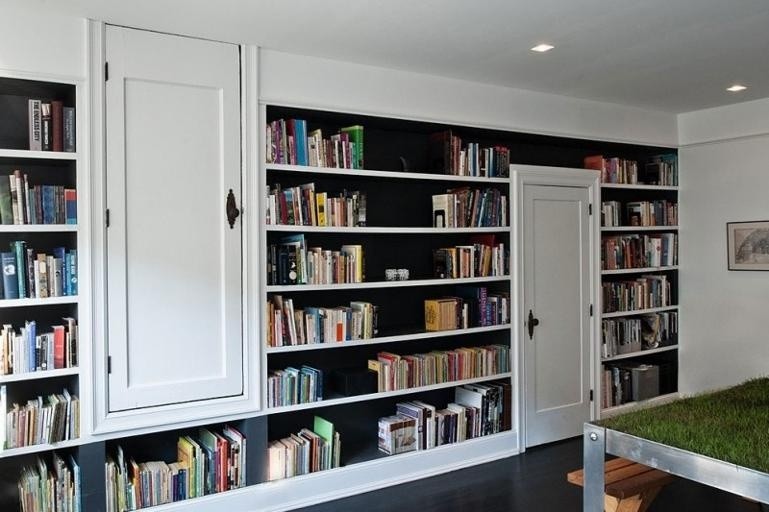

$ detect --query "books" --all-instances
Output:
[368,344,509,391]
[267,368,323,410]
[1,90,84,512]
[434,234,511,278]
[267,296,378,348]
[266,183,366,226]
[431,186,507,228]
[425,287,510,331]
[267,417,340,481]
[266,119,364,169]
[583,148,681,409]
[441,131,509,178]
[102,423,249,511]
[267,235,362,284]
[378,381,511,454]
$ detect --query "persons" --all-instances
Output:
[741,241,753,263]
[755,239,769,255]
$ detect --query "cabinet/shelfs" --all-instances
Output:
[0,373,511,510]
[257,99,513,411]
[596,139,678,410]
[0,74,88,452]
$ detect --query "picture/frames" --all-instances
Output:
[726,220,769,271]
